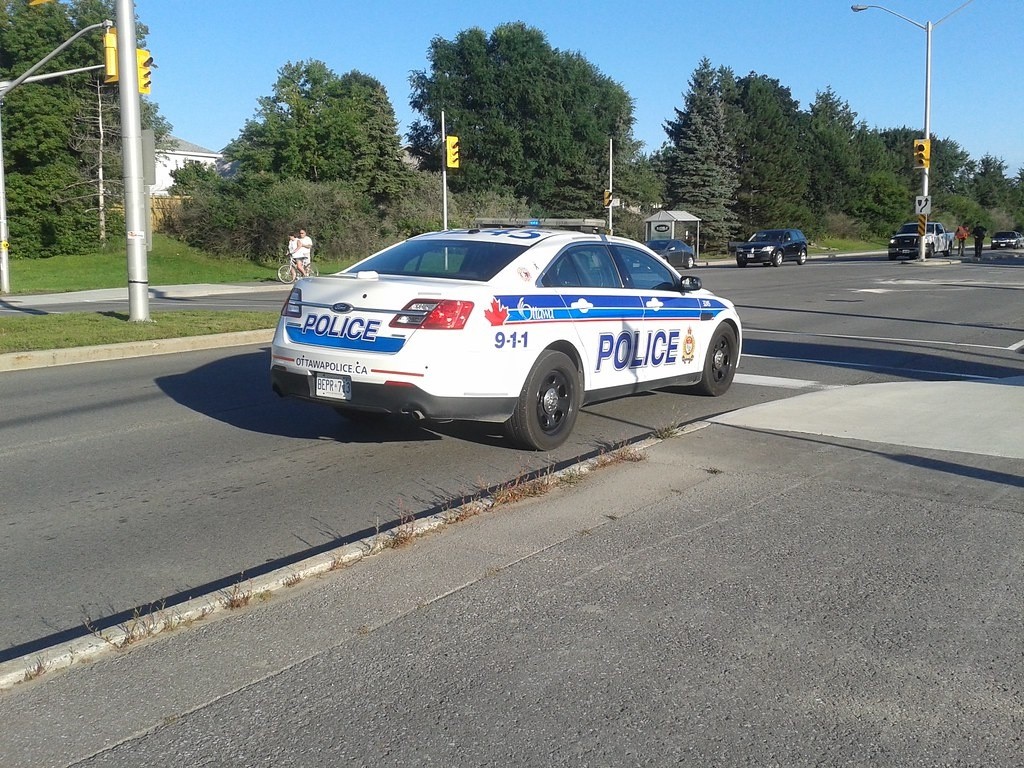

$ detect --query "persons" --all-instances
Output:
[953,222,970,257]
[970,221,987,257]
[296,229,313,275]
[285,231,308,282]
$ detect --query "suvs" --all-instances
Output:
[736,229,809,267]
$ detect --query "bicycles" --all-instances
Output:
[278,255,322,284]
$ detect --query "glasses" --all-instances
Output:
[300,233,304,234]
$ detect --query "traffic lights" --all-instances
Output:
[446,136,459,168]
[136,48,154,94]
[604,192,613,207]
[918,143,926,165]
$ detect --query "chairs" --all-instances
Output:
[557,254,589,286]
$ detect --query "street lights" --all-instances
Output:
[851,0,979,260]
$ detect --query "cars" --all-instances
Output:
[269,219,742,448]
[644,239,697,270]
[990,231,1024,250]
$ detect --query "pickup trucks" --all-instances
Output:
[888,221,955,260]
